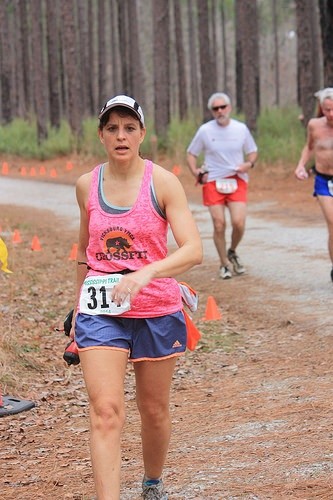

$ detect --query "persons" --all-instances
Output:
[69,95,204,500]
[294,88,333,281]
[186,92,258,280]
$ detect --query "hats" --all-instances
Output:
[99,95,145,128]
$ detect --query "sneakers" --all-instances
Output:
[219,265,232,278]
[140,478,164,500]
[227,249,245,274]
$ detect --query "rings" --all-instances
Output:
[127,287,131,292]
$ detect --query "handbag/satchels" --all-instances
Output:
[215,178,237,194]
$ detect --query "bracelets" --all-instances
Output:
[246,159,254,167]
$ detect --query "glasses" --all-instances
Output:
[212,104,227,110]
